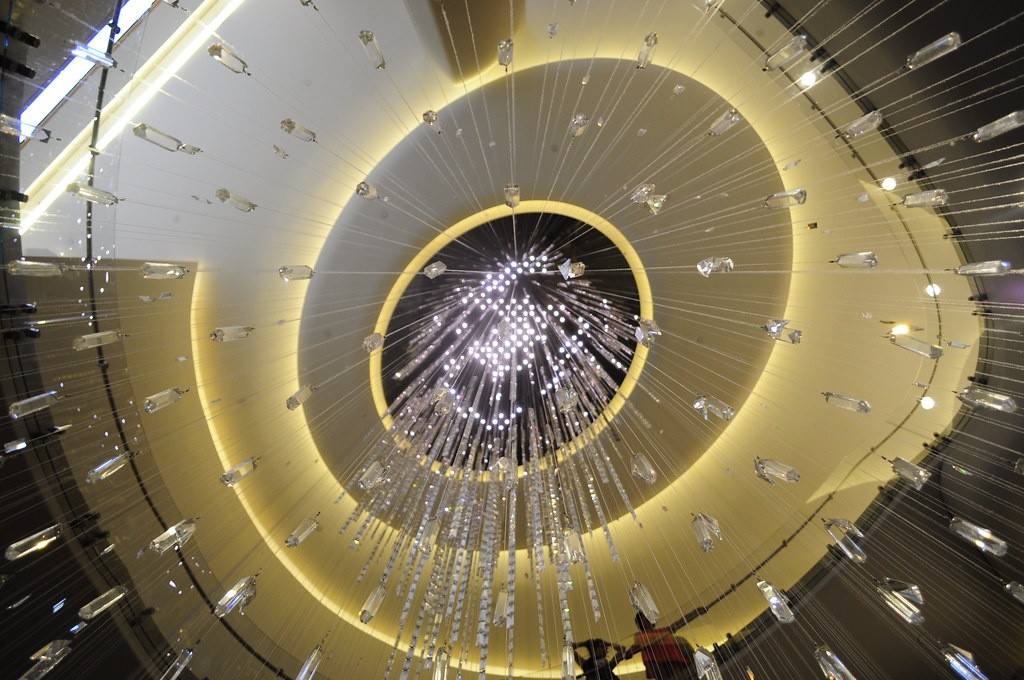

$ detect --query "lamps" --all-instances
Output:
[0,0,1024,680]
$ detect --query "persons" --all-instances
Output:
[625,606,708,680]
[573,638,627,680]
